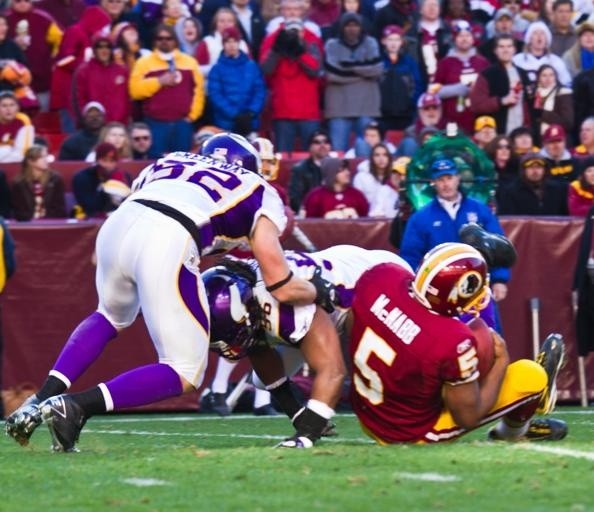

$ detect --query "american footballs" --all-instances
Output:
[467,318,494,382]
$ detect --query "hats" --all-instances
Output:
[417,93,441,108]
[475,116,497,131]
[430,160,458,180]
[544,124,566,143]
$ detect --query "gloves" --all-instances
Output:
[309,266,340,313]
[275,405,339,448]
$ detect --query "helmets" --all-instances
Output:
[201,266,263,362]
[250,137,279,181]
[410,241,491,318]
[201,132,262,176]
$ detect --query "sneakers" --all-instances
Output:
[38,394,88,453]
[458,222,518,269]
[199,384,229,417]
[534,334,565,417]
[488,416,570,442]
[4,394,43,446]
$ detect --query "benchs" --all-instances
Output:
[1,109,405,220]
